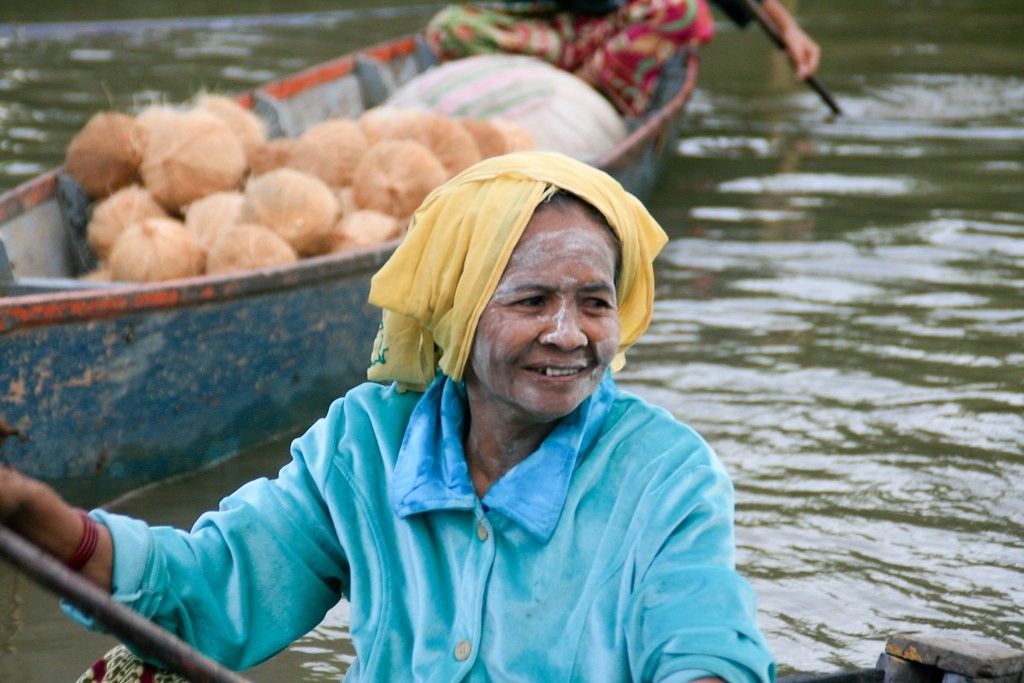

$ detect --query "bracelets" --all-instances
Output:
[63,510,98,572]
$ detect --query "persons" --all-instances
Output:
[424,0,822,120]
[0,149,778,683]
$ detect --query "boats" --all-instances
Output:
[0,25,701,531]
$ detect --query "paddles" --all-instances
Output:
[743,0,846,116]
